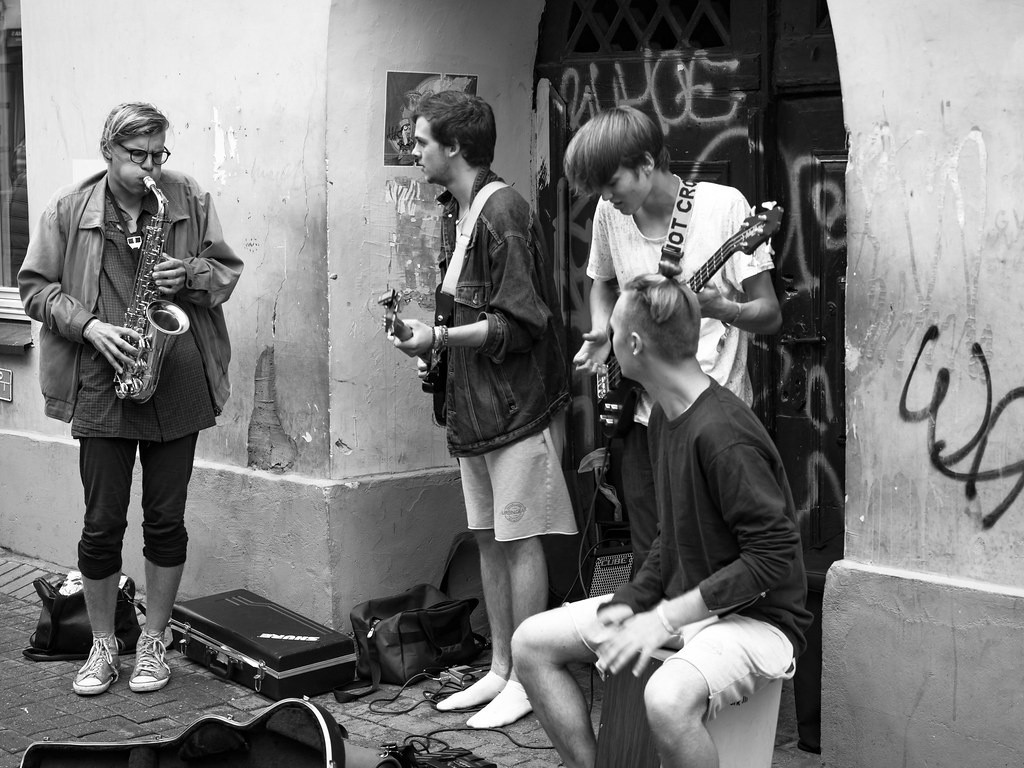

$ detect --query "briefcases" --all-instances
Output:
[170,589,357,702]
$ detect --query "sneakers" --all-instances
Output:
[129,630,171,692]
[73,630,120,693]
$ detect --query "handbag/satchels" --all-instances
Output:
[351,584,478,686]
[22,573,142,662]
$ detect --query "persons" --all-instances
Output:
[385,92,579,728]
[508,244,816,768]
[17,103,246,694]
[564,103,783,574]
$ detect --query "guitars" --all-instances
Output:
[378,284,451,428]
[567,200,783,466]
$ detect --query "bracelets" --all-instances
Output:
[720,303,741,330]
[431,325,448,349]
[656,605,683,635]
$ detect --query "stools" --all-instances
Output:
[595,647,783,768]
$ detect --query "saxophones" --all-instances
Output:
[113,175,191,405]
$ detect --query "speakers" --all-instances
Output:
[584,545,634,602]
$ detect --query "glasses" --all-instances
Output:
[114,141,170,166]
